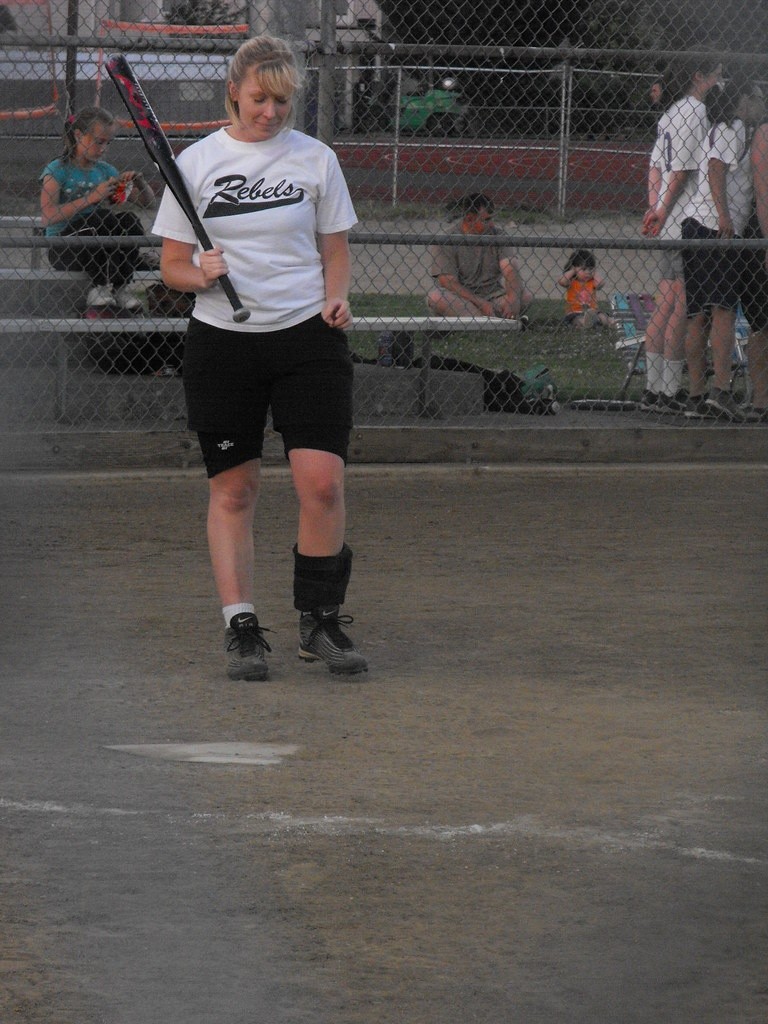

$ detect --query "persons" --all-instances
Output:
[559,250,608,329]
[151,35,370,680]
[37,106,157,312]
[680,73,768,424]
[641,51,726,414]
[426,192,535,330]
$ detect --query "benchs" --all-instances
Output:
[0,267,163,314]
[0,315,522,424]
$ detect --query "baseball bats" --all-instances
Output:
[103,53,254,325]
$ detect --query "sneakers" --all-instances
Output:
[298,604,368,675]
[223,612,278,681]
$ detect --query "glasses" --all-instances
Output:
[476,213,492,223]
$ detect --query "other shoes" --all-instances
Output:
[115,281,143,312]
[86,283,115,307]
[639,387,768,423]
[521,315,534,331]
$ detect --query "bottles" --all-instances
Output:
[378,330,394,366]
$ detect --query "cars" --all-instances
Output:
[399,90,475,137]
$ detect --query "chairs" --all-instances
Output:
[732,297,753,403]
[611,291,749,403]
